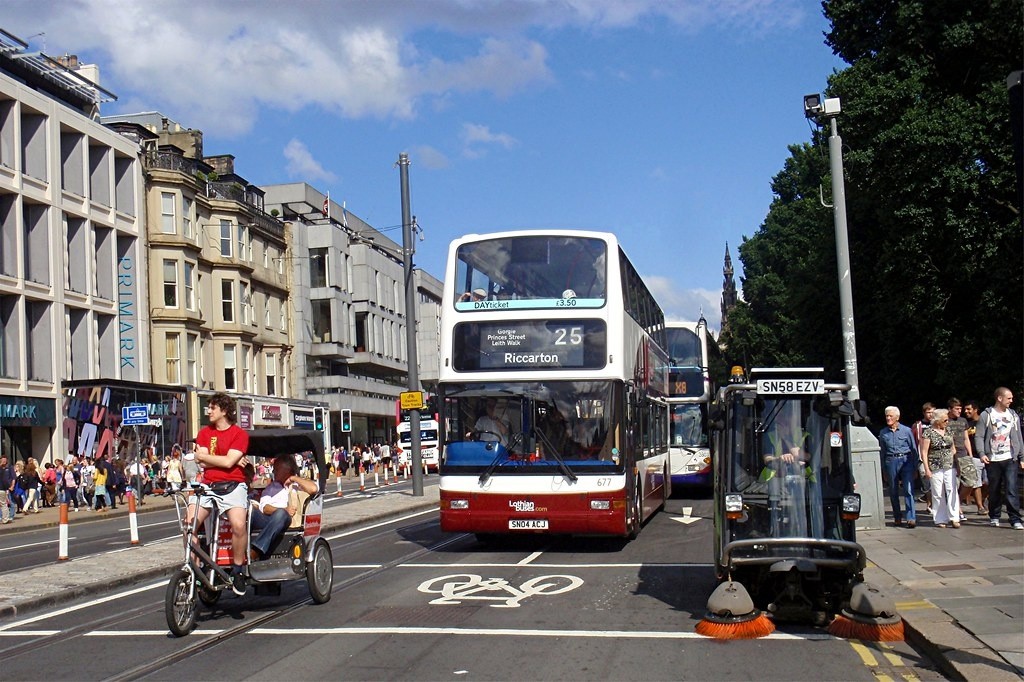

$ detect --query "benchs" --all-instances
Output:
[245,487,306,536]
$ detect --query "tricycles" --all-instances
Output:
[151,428,335,638]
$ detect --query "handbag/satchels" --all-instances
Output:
[329,458,375,477]
[210,480,239,494]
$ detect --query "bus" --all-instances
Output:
[396,396,458,476]
[427,229,672,548]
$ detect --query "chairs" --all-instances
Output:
[495,399,523,454]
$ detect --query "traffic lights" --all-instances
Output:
[313,407,325,432]
[341,409,352,432]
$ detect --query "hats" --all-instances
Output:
[562,289,576,299]
[473,288,486,299]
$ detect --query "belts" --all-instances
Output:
[887,452,911,457]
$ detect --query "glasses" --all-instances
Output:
[940,419,949,423]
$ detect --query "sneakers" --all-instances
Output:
[228,569,246,596]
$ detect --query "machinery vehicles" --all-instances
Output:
[645,320,729,487]
[694,349,906,644]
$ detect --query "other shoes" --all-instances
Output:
[951,520,960,527]
[907,520,917,528]
[1010,522,1024,530]
[960,514,967,521]
[250,547,261,562]
[977,508,987,515]
[3,499,146,524]
[919,496,928,503]
[937,523,946,528]
[894,517,901,525]
[989,518,1000,527]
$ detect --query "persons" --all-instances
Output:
[181,393,249,596]
[880,406,921,527]
[0,443,196,524]
[253,440,398,483]
[250,454,318,560]
[457,289,487,302]
[975,387,1024,530]
[912,397,990,528]
[562,288,577,298]
[465,397,509,446]
[758,405,819,535]
[574,419,597,447]
